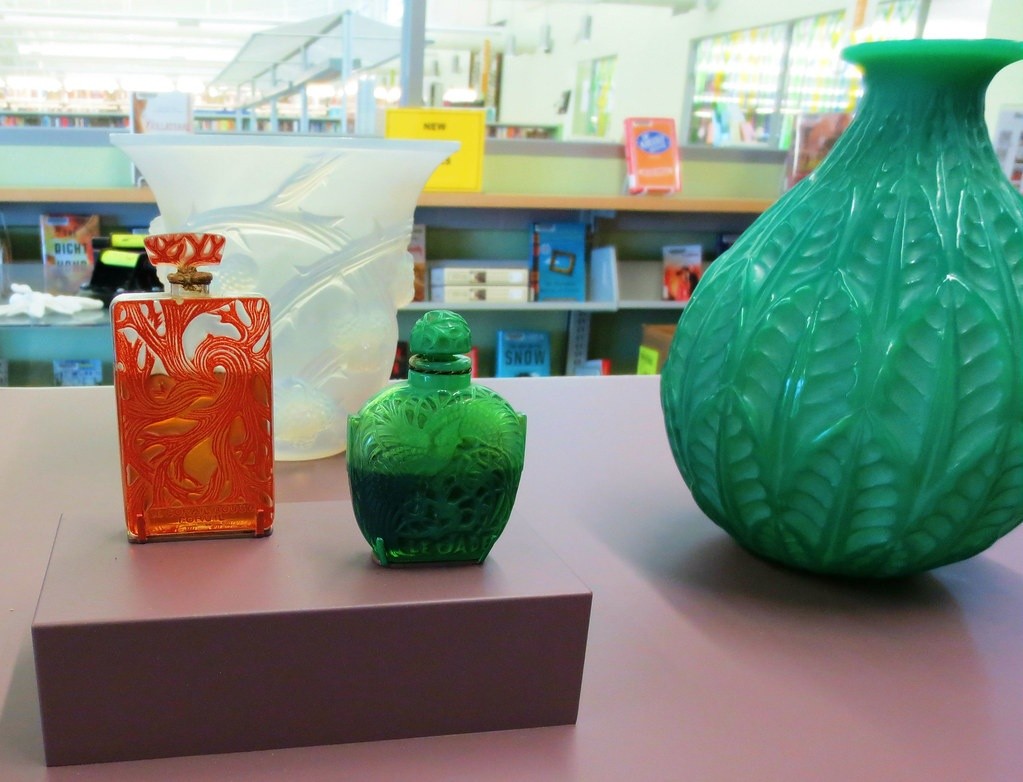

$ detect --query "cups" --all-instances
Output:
[109,128,462,459]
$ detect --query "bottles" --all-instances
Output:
[344,307,532,567]
[113,228,278,541]
[655,33,1023,582]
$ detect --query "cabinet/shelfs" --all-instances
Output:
[0,126,787,360]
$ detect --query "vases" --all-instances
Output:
[660,40,1023,583]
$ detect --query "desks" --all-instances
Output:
[0,374,1023,782]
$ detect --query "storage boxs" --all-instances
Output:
[640,324,676,374]
